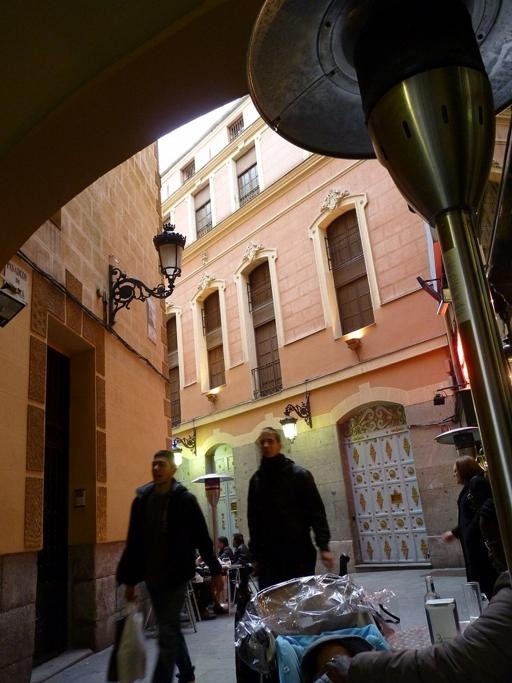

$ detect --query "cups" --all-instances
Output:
[461,581,487,623]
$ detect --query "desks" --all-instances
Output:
[386,621,470,651]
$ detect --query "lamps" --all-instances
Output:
[109,224,186,327]
[345,337,361,350]
[434,386,459,405]
[172,428,196,467]
[280,394,312,443]
[207,393,218,401]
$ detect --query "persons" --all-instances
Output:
[191,552,227,621]
[115,448,230,683]
[311,642,352,683]
[230,532,249,576]
[246,426,334,595]
[324,495,511,682]
[441,455,502,600]
[217,535,232,559]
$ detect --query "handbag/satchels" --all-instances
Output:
[108,602,145,683]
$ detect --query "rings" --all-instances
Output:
[331,657,334,661]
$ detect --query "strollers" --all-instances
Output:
[236,554,394,682]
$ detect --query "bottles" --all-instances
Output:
[423,575,440,603]
